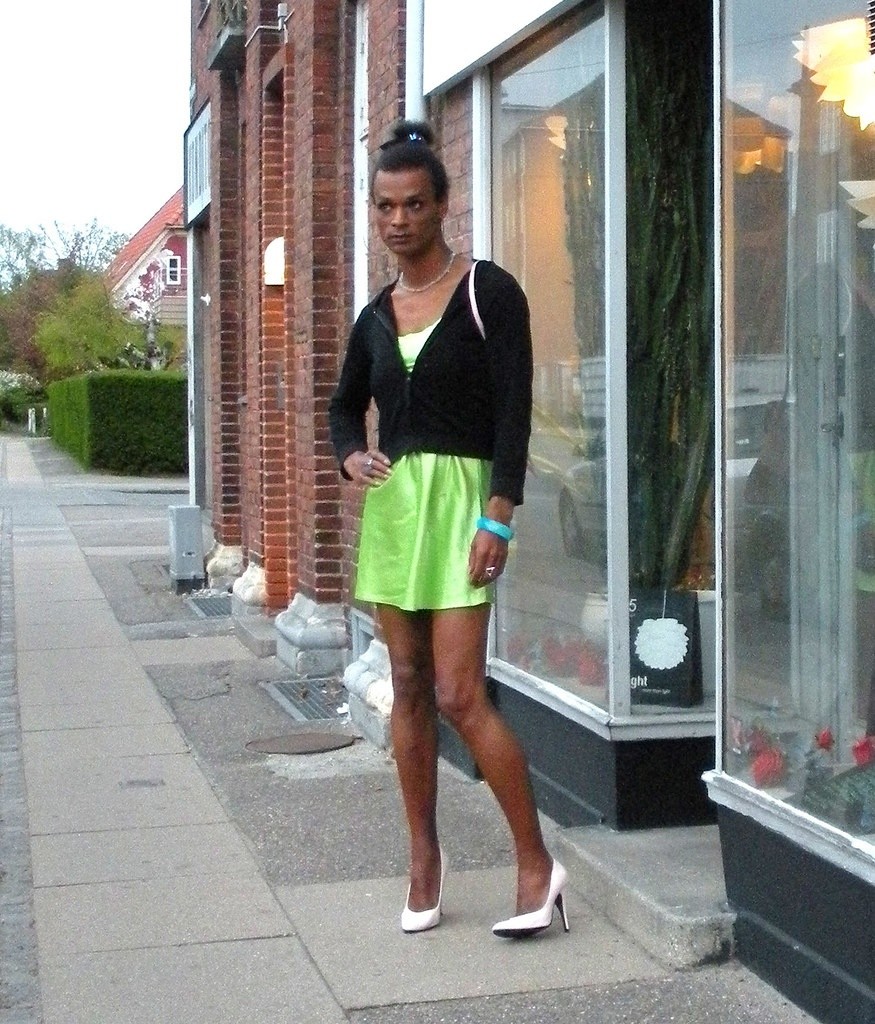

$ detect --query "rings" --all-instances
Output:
[365,457,375,468]
[486,566,497,578]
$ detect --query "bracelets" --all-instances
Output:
[477,515,515,542]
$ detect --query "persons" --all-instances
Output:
[329,122,570,937]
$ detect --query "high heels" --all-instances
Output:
[492,857,570,938]
[401,843,444,932]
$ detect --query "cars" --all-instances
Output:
[559,392,779,561]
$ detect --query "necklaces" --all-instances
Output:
[398,252,456,294]
